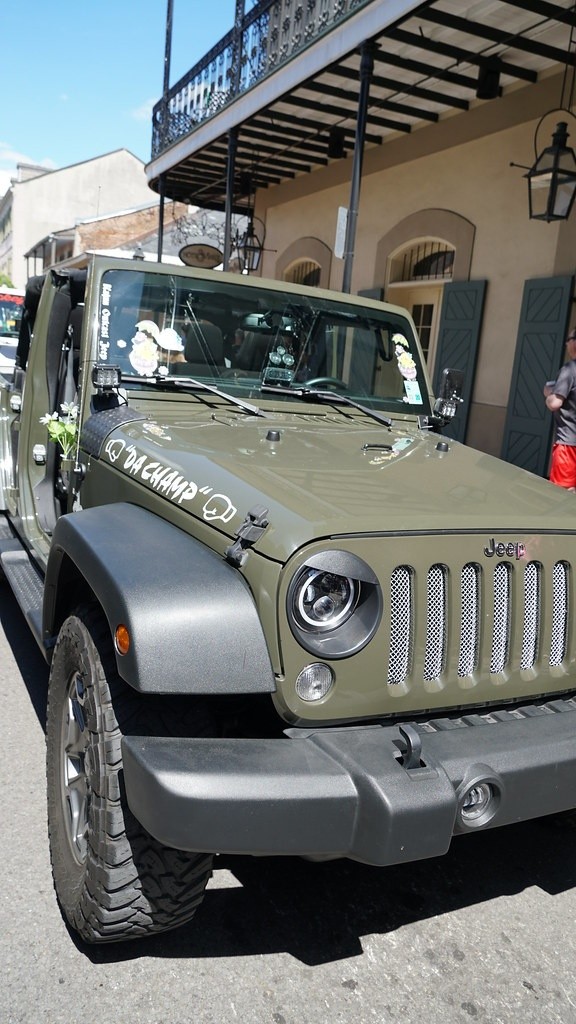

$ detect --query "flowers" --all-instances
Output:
[39,402,78,459]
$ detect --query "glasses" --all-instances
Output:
[566,337,576,342]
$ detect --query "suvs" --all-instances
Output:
[0,252,576,941]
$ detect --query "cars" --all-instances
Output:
[0,285,34,390]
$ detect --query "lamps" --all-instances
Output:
[234,146,267,270]
[521,3,576,223]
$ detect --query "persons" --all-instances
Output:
[543,325,576,493]
[151,324,188,364]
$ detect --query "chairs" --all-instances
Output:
[231,332,305,383]
[172,323,227,379]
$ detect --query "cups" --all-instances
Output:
[545,380,556,395]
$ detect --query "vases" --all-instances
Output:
[59,458,73,488]
